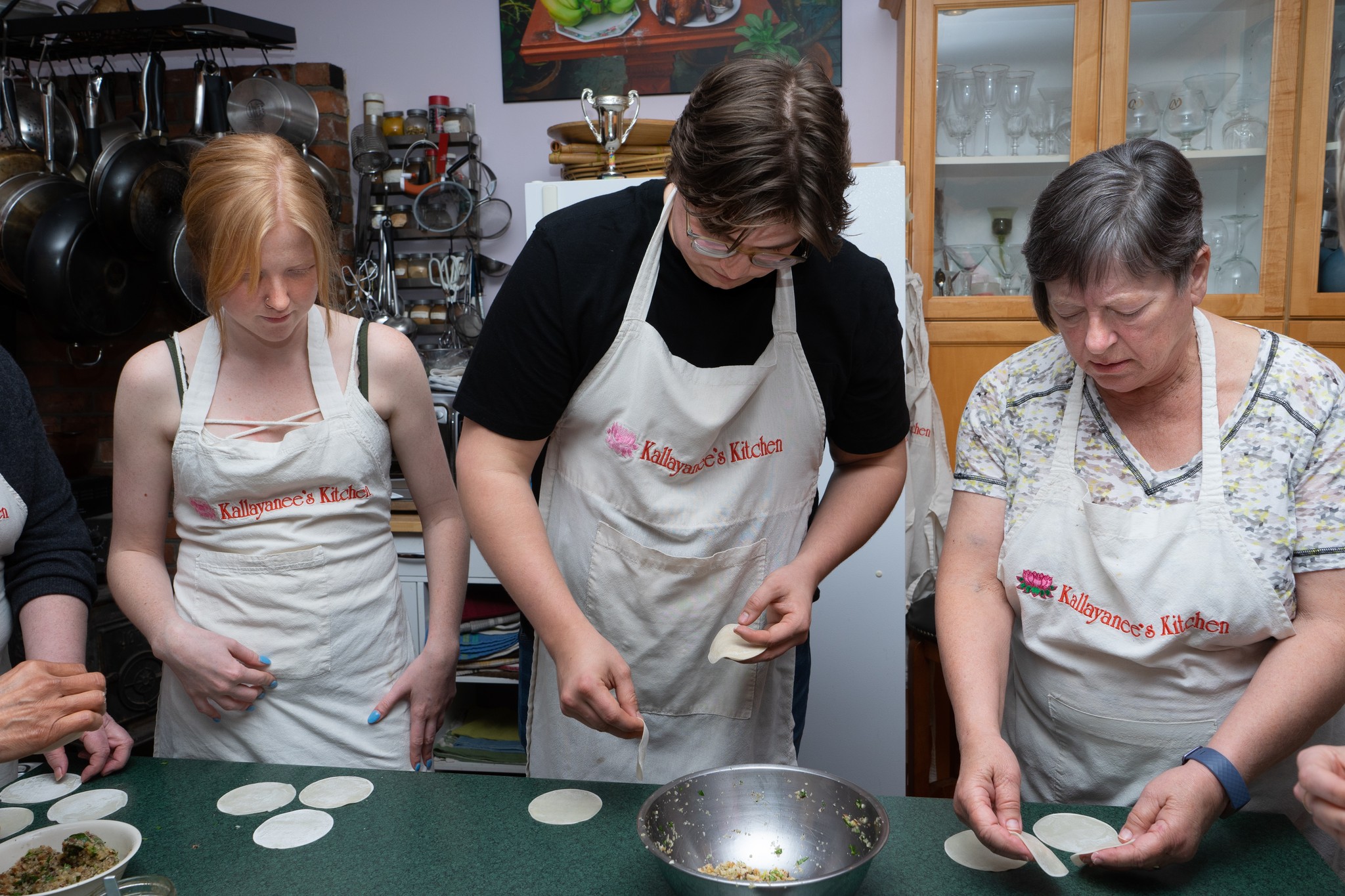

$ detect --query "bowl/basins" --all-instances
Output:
[637,763,891,896]
[0,819,143,896]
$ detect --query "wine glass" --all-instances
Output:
[937,64,1269,158]
[943,206,1034,296]
[1199,214,1261,295]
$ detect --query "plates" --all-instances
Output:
[547,118,678,147]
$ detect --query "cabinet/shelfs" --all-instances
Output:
[389,533,540,774]
[353,103,482,372]
[874,0,1345,322]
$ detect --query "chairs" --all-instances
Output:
[801,35,833,81]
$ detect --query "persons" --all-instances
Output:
[0,343,134,790]
[1294,745,1345,850]
[935,139,1345,875]
[451,53,913,782]
[107,131,472,771]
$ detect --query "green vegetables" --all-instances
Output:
[11,833,119,896]
[768,791,873,883]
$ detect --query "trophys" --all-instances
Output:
[582,88,640,180]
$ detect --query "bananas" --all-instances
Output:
[540,0,635,27]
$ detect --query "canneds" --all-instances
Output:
[361,92,475,325]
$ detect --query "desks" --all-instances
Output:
[520,0,779,93]
[0,754,1345,896]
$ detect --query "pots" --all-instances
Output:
[0,53,342,369]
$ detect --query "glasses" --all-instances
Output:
[685,201,813,269]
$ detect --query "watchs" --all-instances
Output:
[1182,746,1251,818]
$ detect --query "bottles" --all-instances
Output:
[89,876,176,896]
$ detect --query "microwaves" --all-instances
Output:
[389,391,463,500]
